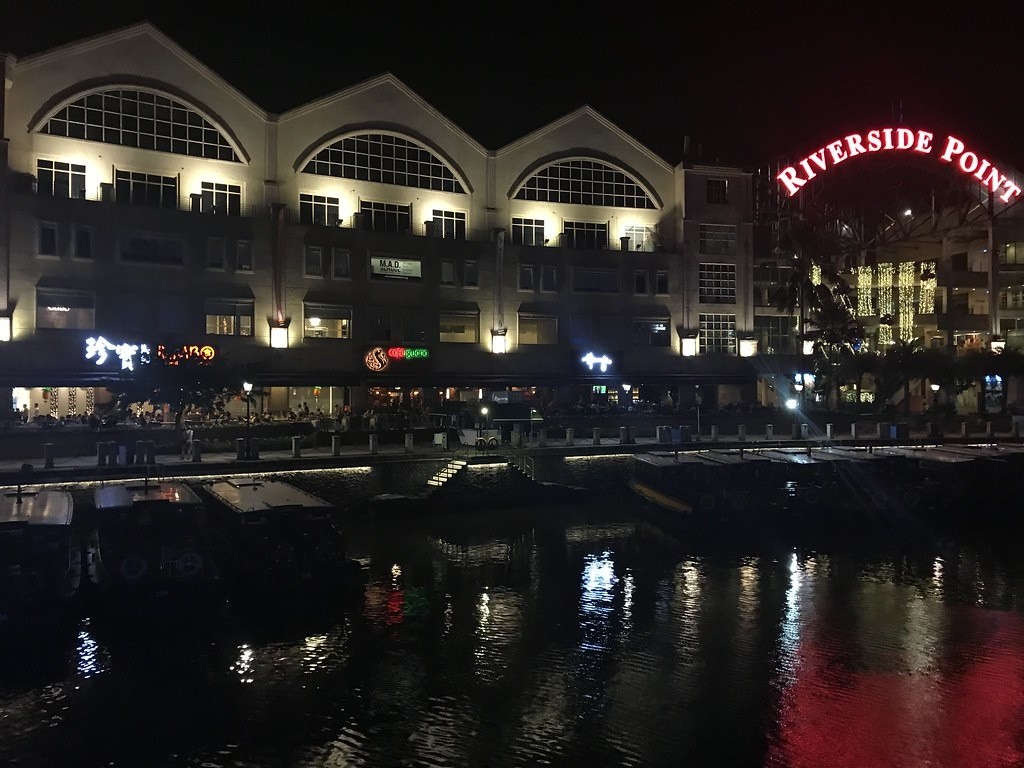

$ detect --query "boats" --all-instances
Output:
[93,478,214,606]
[0,483,83,598]
[628,444,1024,530]
[200,478,349,584]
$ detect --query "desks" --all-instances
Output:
[493,418,545,445]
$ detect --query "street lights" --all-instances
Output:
[243,382,253,458]
[481,408,488,451]
[696,385,700,433]
[622,384,631,444]
[930,381,940,434]
[794,382,803,424]
[529,407,538,437]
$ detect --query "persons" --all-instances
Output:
[186,401,352,435]
[962,336,987,351]
[13,404,164,426]
[179,424,194,460]
[576,396,786,418]
[362,395,433,433]
